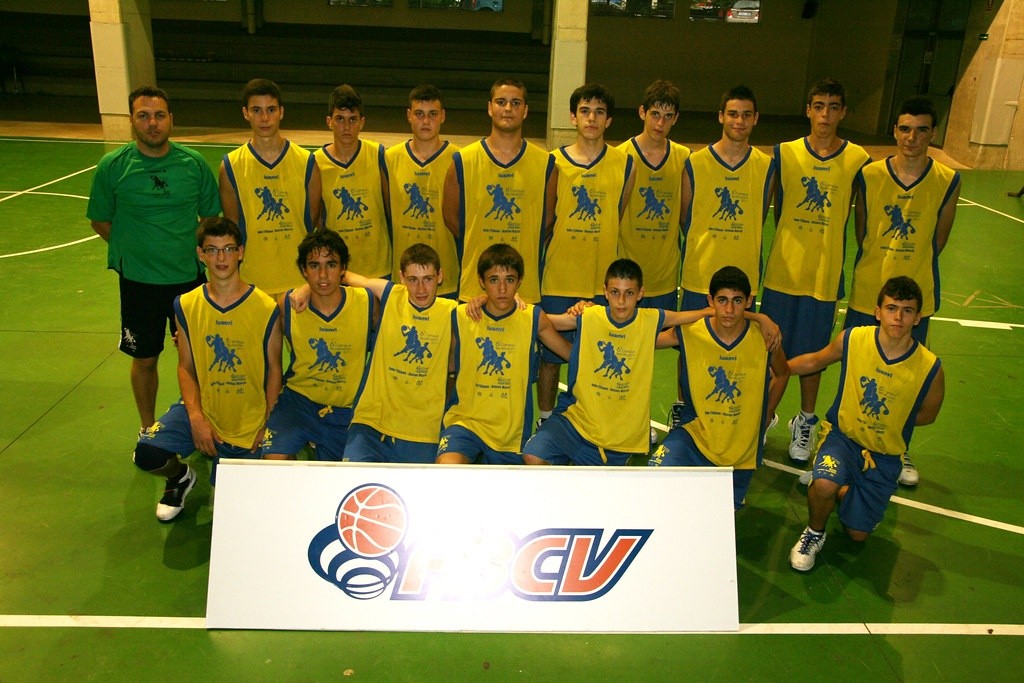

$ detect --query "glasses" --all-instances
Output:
[202,247,239,254]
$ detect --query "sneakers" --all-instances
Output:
[762,413,778,446]
[791,525,827,571]
[536,417,546,431]
[156,463,197,521]
[899,452,918,486]
[133,426,150,462]
[667,400,685,432]
[788,411,819,461]
[798,470,814,485]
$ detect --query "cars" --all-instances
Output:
[589,0,761,23]
[329,0,505,13]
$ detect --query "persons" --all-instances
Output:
[289,244,527,464]
[798,96,960,489]
[132,218,283,521]
[218,77,321,309]
[769,276,945,571]
[441,76,558,305]
[566,266,790,514]
[385,85,459,297]
[615,80,691,445]
[466,259,782,465]
[671,87,777,429]
[536,84,636,433]
[436,244,574,464]
[87,87,221,460]
[760,79,873,460]
[315,85,395,280]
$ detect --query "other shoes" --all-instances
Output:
[650,426,658,443]
